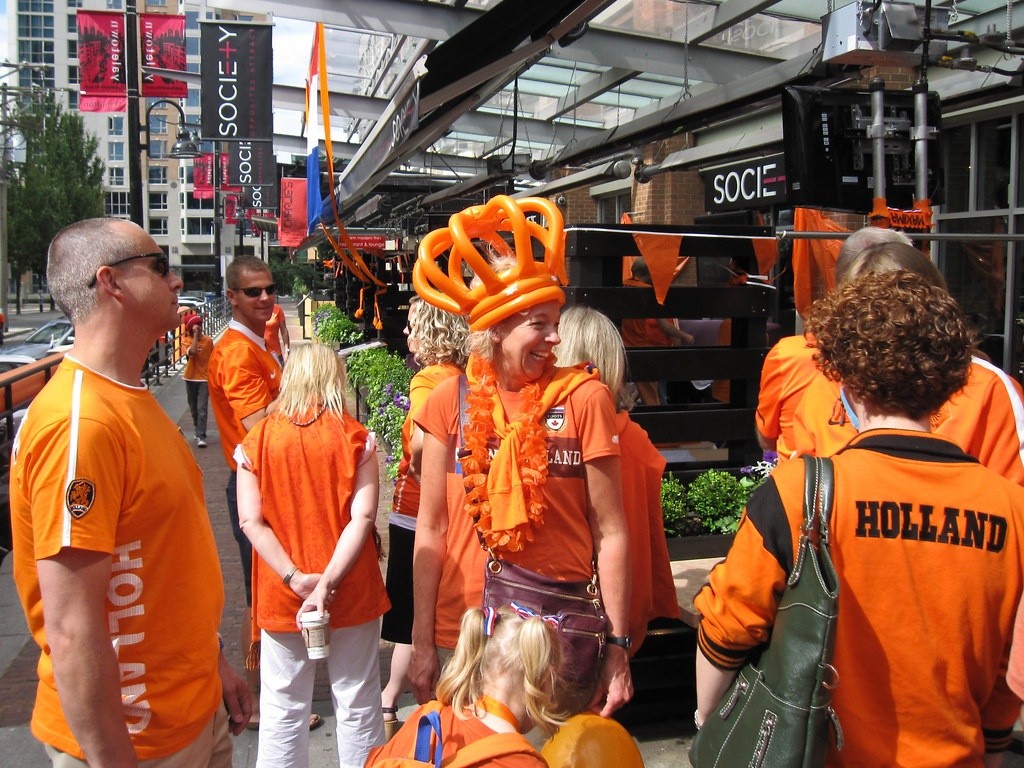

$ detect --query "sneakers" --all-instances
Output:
[196,435,207,447]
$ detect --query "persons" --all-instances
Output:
[690,269,1024,768]
[233,343,392,768]
[209,253,324,731]
[264,303,290,369]
[9,215,251,768]
[623,257,694,406]
[363,607,561,768]
[382,191,1024,738]
[181,314,214,447]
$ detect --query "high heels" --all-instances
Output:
[382,705,398,743]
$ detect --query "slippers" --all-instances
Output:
[247,712,320,731]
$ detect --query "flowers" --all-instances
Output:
[346,348,415,485]
[308,302,358,347]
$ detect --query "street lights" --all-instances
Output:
[213,194,248,297]
[239,218,259,254]
[127,100,203,233]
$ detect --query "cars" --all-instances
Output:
[0,316,76,520]
[149,291,221,366]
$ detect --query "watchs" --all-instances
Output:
[605,635,633,649]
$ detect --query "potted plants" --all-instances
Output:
[654,470,748,561]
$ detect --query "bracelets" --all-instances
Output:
[281,565,299,585]
[693,710,705,729]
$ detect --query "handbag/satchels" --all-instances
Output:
[480,555,609,718]
[620,616,716,745]
[689,453,839,768]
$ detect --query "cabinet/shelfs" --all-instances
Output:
[562,226,791,487]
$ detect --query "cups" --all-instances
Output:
[299,610,331,660]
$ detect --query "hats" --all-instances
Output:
[183,313,202,331]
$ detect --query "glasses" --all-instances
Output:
[87,250,170,290]
[231,284,280,298]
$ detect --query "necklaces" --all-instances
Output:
[285,406,324,426]
[460,357,549,553]
